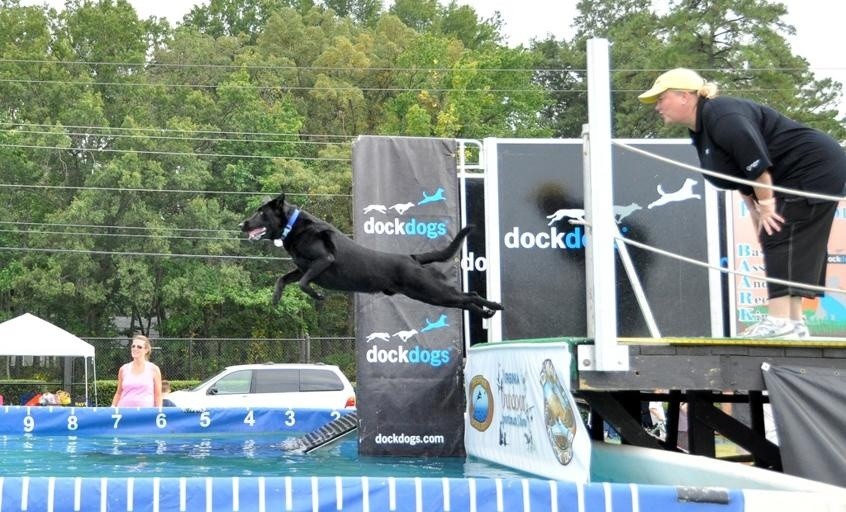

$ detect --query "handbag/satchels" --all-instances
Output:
[55,389,72,405]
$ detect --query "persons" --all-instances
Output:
[110,334,162,408]
[637,67,846,340]
[161,380,176,407]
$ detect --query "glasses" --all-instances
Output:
[131,342,145,351]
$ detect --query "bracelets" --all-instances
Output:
[755,196,776,207]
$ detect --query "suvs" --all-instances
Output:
[161,359,356,415]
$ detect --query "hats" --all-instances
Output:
[637,66,708,103]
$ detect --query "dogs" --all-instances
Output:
[237,191,506,320]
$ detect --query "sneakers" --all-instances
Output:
[737,316,811,341]
